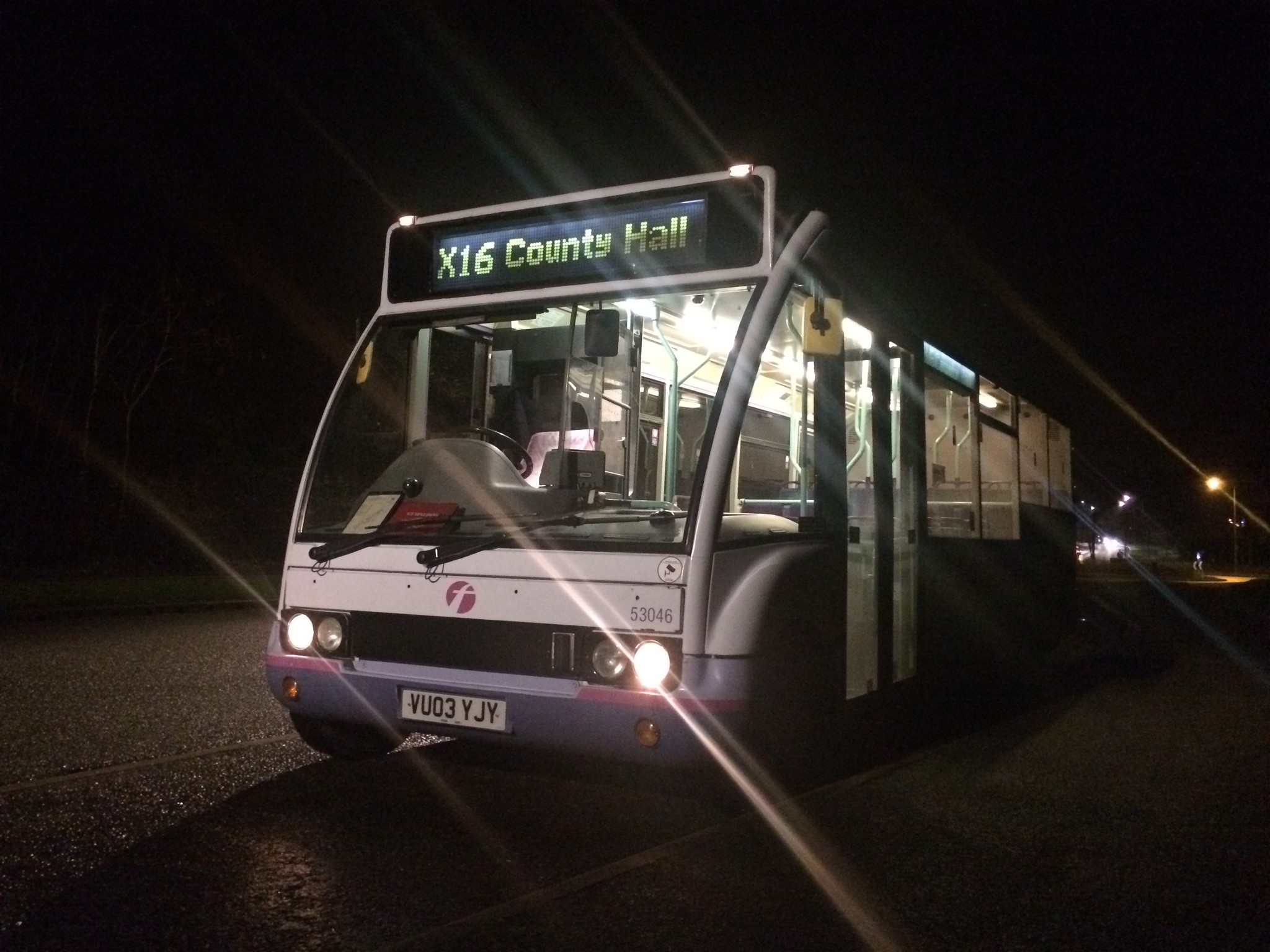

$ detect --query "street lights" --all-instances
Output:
[1206,475,1238,577]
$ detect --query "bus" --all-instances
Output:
[262,161,1077,769]
